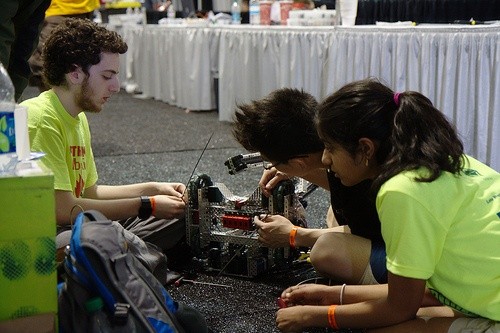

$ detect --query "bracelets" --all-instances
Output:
[289,225,299,247]
[340,283,346,304]
[328,305,337,329]
[149,196,156,217]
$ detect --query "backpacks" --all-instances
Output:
[59,210,207,333]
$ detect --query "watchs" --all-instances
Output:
[138,194,152,221]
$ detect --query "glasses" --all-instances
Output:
[263,156,309,170]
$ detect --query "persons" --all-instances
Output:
[0,0,52,104]
[18,16,188,286]
[28,0,100,93]
[275,77,500,333]
[231,85,384,288]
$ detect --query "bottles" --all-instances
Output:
[260,0,272,24]
[249,0,260,24]
[280,0,293,25]
[231,0,241,25]
[126,3,147,24]
[167,4,175,19]
[0,61,18,172]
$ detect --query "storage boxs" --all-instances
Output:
[0,158,59,333]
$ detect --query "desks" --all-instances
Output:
[105,24,500,173]
